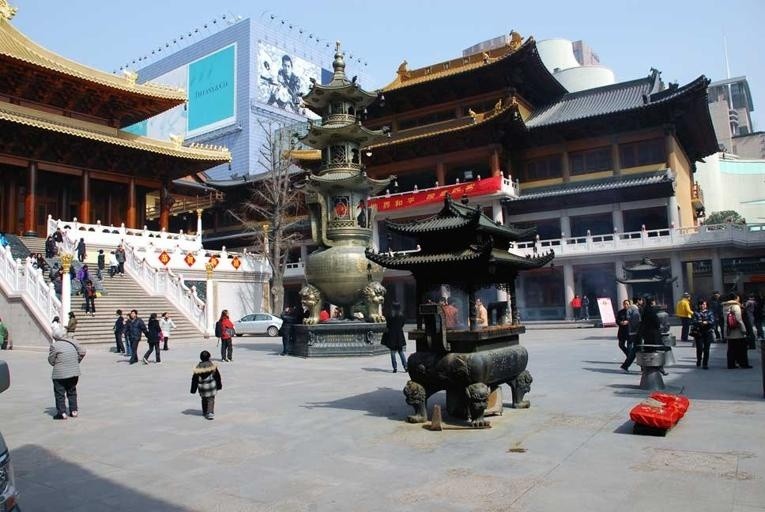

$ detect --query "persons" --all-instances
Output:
[443,297,459,330]
[190,350,222,419]
[614,287,764,372]
[319,307,329,321]
[30,227,177,364]
[572,295,582,319]
[277,55,301,114]
[475,297,489,327]
[0,317,8,350]
[48,327,87,420]
[381,299,409,372]
[581,295,590,320]
[219,310,236,362]
[439,297,447,307]
[277,305,297,356]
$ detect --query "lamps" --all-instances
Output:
[112,8,236,78]
[259,9,369,69]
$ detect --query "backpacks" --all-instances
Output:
[215,322,222,337]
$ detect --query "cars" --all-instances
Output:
[231,313,283,338]
[1,362,23,512]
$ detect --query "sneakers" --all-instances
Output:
[70,409,78,416]
[203,411,215,419]
[53,412,67,420]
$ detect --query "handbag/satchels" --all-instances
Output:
[689,322,704,337]
[727,312,740,331]
